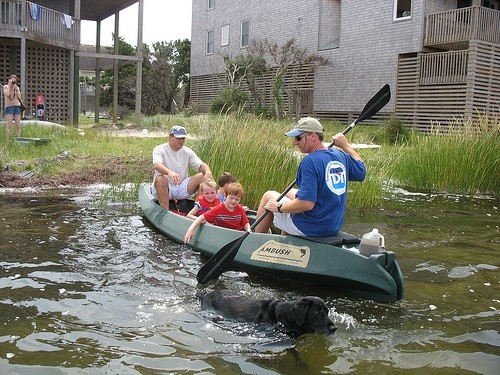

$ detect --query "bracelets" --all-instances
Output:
[16,90,18,91]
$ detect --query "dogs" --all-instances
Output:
[194,278,338,336]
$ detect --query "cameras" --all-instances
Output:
[12,81,15,84]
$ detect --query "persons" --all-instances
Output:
[217,172,250,211]
[187,180,221,221]
[255,117,366,238]
[4,75,22,137]
[185,182,251,243]
[153,126,214,209]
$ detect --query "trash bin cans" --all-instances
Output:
[36,95,45,120]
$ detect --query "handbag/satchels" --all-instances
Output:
[20,104,26,112]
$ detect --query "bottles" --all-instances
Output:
[360,227,385,255]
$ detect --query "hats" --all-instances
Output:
[284,117,325,137]
[169,126,188,138]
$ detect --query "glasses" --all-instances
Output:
[295,134,311,141]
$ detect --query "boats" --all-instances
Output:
[138,182,405,304]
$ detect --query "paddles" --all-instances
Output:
[196,83,391,285]
[20,150,68,179]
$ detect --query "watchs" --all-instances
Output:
[277,203,282,213]
